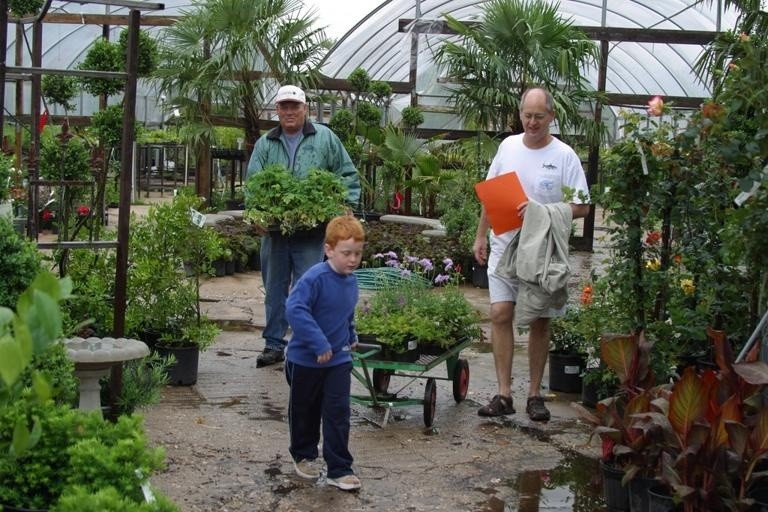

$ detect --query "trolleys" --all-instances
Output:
[330,328,484,432]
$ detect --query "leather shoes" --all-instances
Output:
[256,349,286,366]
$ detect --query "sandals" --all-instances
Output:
[527,397,549,419]
[478,395,516,415]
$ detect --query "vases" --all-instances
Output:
[138,326,183,351]
[354,212,383,221]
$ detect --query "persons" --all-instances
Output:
[244,85,362,367]
[285,216,365,491]
[472,87,592,421]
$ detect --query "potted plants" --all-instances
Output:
[243,164,356,237]
[38,177,57,230]
[518,310,589,393]
[570,327,768,512]
[459,218,488,288]
[158,280,213,385]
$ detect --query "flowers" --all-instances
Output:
[576,281,617,400]
[353,251,488,361]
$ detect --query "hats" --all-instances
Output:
[275,85,307,104]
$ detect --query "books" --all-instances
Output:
[474,171,529,235]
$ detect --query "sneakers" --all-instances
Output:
[327,473,361,489]
[294,460,320,479]
